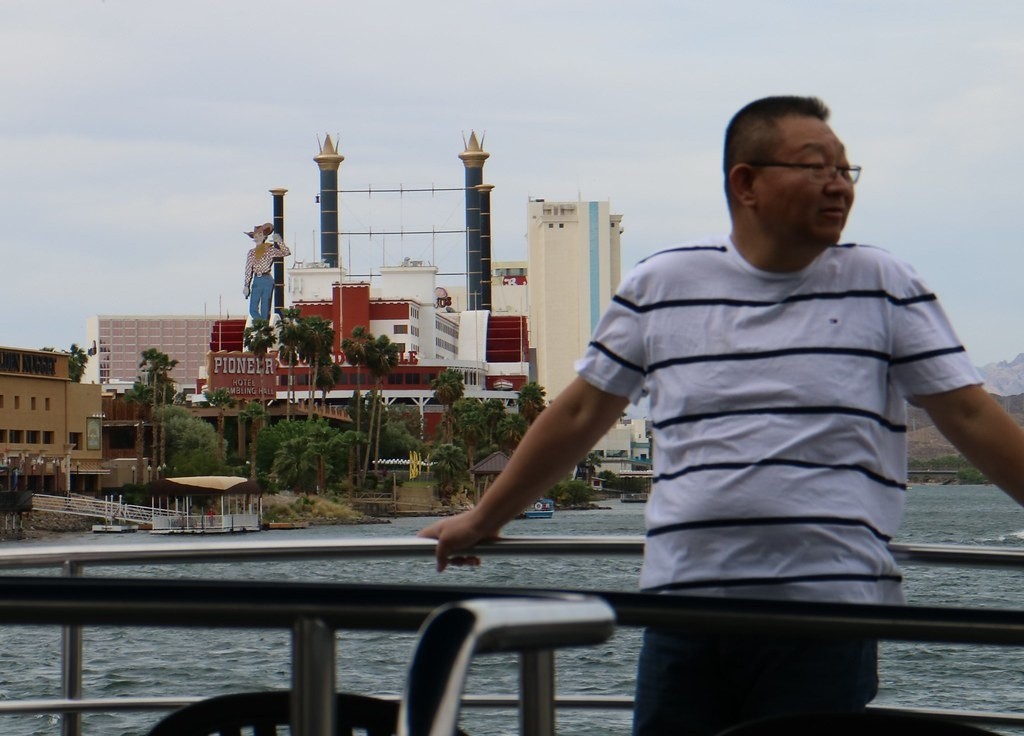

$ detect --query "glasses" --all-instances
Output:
[747,163,862,185]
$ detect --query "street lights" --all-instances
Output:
[56,460,59,494]
[30,460,35,493]
[157,465,161,479]
[76,461,79,494]
[132,466,135,485]
[147,465,151,482]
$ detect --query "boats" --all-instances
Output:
[620,493,648,503]
[524,497,555,519]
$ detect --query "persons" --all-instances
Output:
[208,508,216,527]
[419,96,1024,736]
